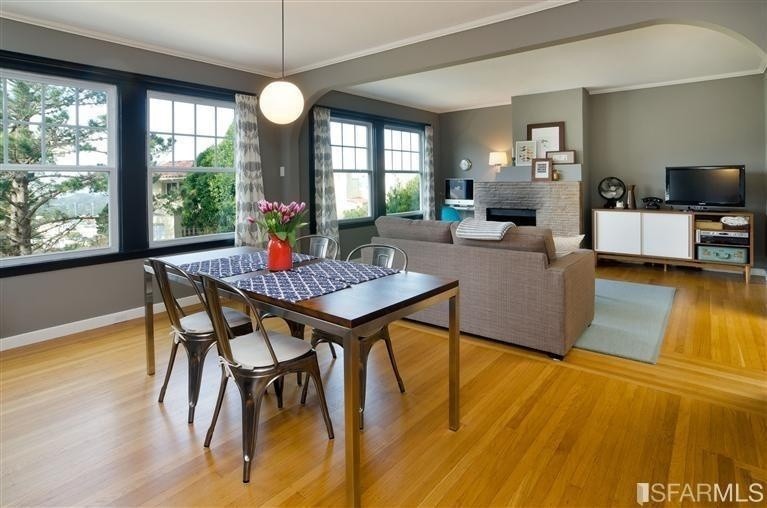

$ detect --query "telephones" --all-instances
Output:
[642,197,662,209]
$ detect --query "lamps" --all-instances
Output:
[258,0,304,125]
[489,152,507,174]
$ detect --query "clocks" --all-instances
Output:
[459,159,472,171]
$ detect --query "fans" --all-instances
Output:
[598,177,627,210]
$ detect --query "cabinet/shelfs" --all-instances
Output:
[591,208,754,284]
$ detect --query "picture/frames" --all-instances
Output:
[527,121,565,152]
[545,151,577,164]
[531,158,553,182]
[515,141,536,167]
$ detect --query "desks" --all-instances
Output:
[143,247,460,508]
[447,205,474,211]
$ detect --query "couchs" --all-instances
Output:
[359,216,595,361]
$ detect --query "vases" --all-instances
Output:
[266,232,293,272]
[626,184,637,210]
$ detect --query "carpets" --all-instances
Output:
[345,257,678,365]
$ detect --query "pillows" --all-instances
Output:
[553,235,585,257]
[450,221,557,263]
[374,215,452,244]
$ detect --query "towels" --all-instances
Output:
[457,217,516,240]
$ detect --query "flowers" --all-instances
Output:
[246,197,310,249]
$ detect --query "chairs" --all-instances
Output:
[438,207,462,220]
[148,257,269,424]
[299,242,407,429]
[196,270,334,481]
[253,234,338,387]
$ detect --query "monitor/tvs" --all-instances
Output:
[443,177,474,210]
[664,164,746,212]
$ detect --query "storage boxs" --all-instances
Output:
[698,246,747,264]
